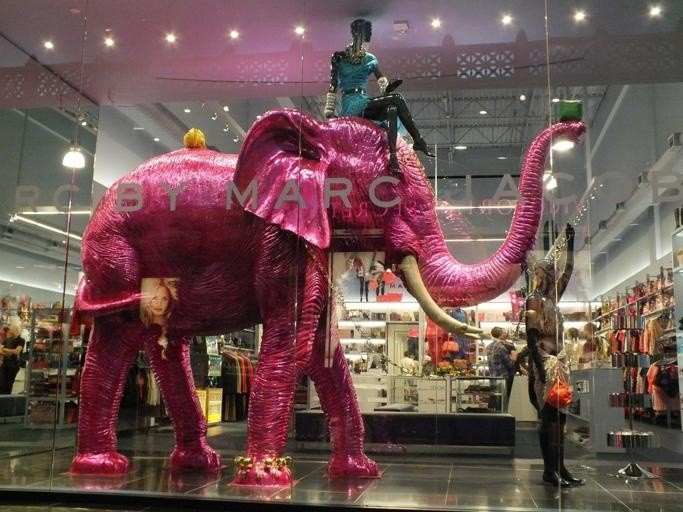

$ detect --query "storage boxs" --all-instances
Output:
[554,100,584,121]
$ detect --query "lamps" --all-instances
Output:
[61,127,86,169]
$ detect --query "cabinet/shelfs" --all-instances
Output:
[590,267,681,429]
[333,300,589,420]
[23,306,86,428]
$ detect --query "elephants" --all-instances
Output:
[68,108,588,490]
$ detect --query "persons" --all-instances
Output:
[400,351,415,377]
[502,330,517,398]
[409,352,419,375]
[525,222,587,488]
[486,327,516,412]
[558,328,580,365]
[516,345,542,431]
[140,279,179,329]
[0,325,25,393]
[579,323,600,362]
[325,19,436,175]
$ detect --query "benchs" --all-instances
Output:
[0,394,26,416]
[292,409,515,458]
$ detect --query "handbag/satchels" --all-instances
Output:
[402,315,410,321]
[390,313,401,321]
[454,359,467,370]
[414,312,419,320]
[443,341,460,352]
[548,379,573,408]
[439,362,449,368]
